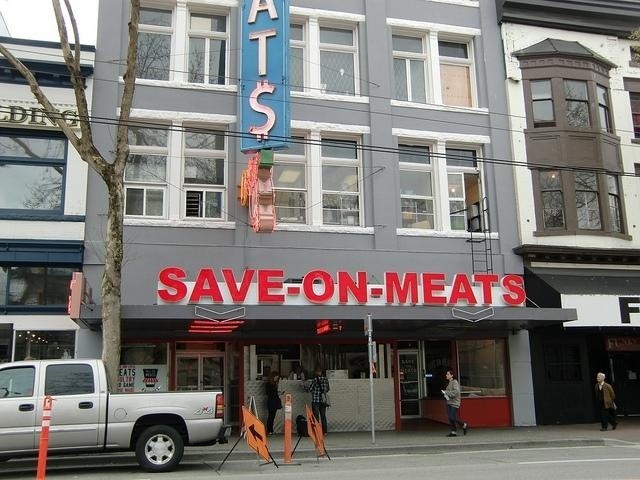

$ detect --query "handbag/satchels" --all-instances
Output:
[321,392,331,407]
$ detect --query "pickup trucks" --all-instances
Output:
[1,358,233,472]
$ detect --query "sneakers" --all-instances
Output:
[266,432,277,437]
[612,421,618,430]
[463,423,468,435]
[600,427,607,431]
[446,433,456,436]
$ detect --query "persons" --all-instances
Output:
[264,371,284,436]
[441,370,469,437]
[286,365,307,381]
[594,373,618,431]
[299,366,330,436]
